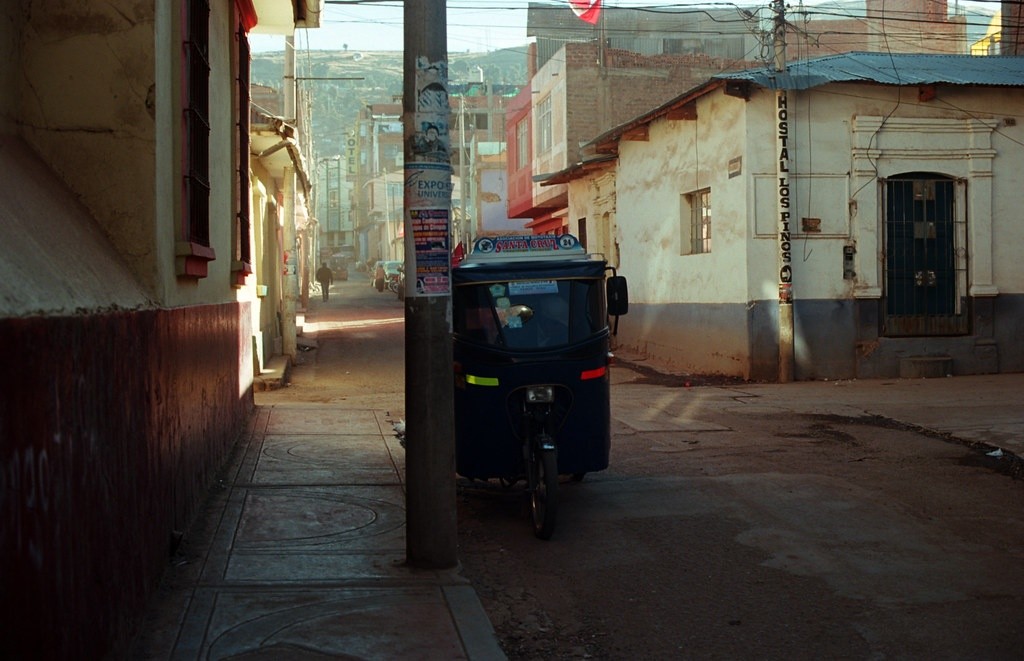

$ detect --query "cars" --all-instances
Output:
[370,261,405,301]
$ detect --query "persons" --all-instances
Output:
[376,265,386,292]
[317,264,330,302]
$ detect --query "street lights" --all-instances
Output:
[363,167,391,260]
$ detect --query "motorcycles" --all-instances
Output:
[454,235,629,542]
